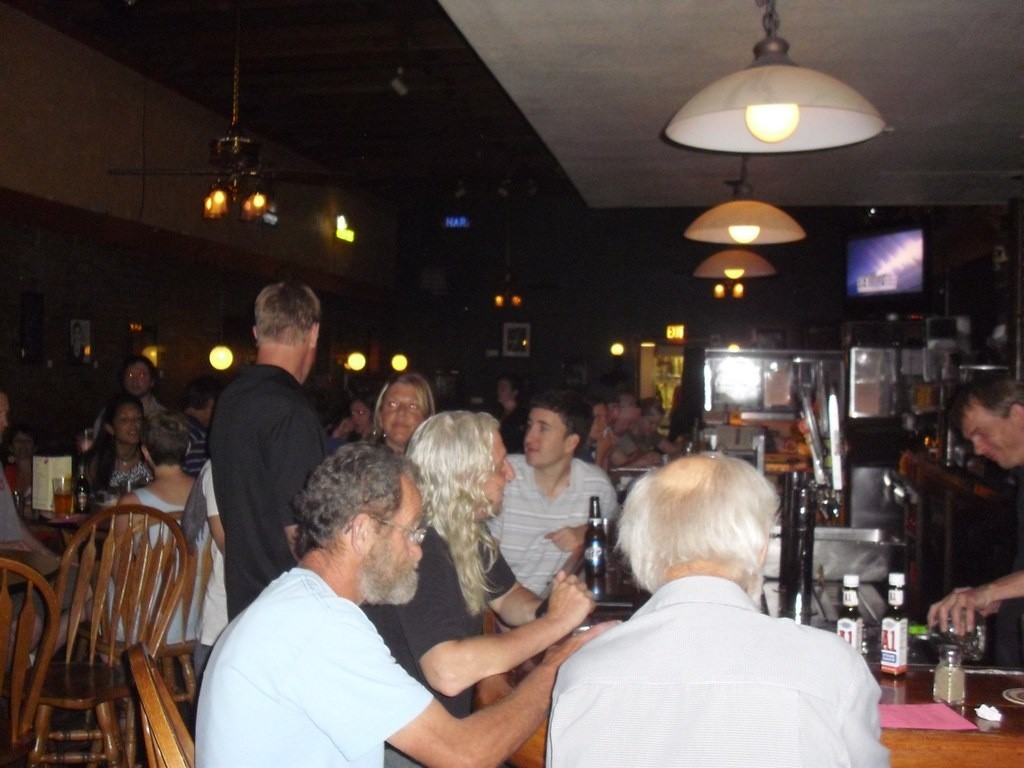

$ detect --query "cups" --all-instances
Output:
[80,431,93,452]
[51,478,72,514]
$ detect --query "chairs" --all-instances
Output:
[0,504,214,768]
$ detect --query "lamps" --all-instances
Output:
[683,154,806,245]
[693,245,776,280]
[492,200,523,309]
[204,0,277,224]
[665,0,886,154]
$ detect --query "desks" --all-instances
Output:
[479,543,1024,768]
[899,448,1021,596]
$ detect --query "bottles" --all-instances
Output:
[74,460,89,511]
[836,574,863,655]
[584,497,607,597]
[880,573,908,680]
[932,645,965,704]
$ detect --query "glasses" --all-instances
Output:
[343,514,427,545]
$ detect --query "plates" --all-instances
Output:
[1002,688,1024,706]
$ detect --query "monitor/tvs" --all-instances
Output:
[843,220,931,298]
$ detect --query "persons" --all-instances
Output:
[208,281,330,623]
[437,372,683,480]
[546,455,891,767]
[331,392,375,441]
[786,418,849,490]
[360,410,597,768]
[488,391,618,595]
[0,356,228,729]
[374,374,433,455]
[926,376,1023,667]
[194,445,622,768]
